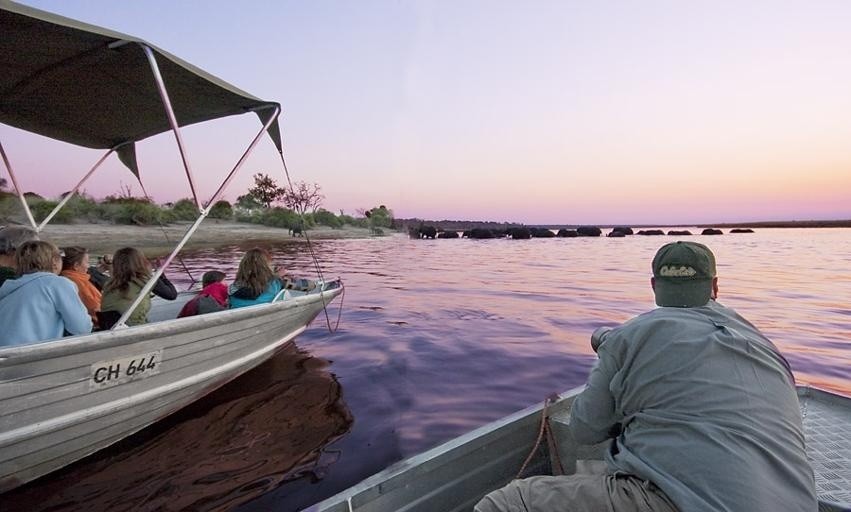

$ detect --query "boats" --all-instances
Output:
[297,366,851,510]
[1,3,343,491]
[0,342,357,511]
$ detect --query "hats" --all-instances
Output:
[651,241,717,307]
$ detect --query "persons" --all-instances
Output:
[228,248,282,309]
[176,271,229,319]
[1,227,177,348]
[472,242,819,512]
